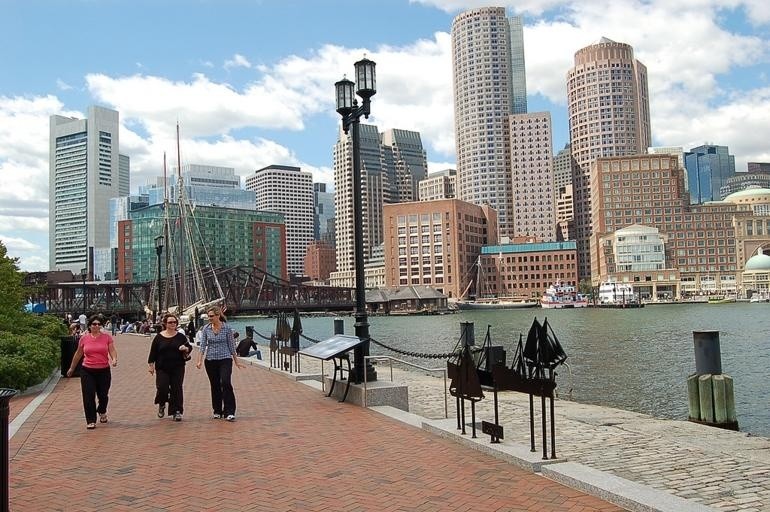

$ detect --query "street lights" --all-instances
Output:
[153,234,165,312]
[332,50,379,383]
[80,267,89,313]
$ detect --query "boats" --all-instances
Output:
[707,294,736,304]
[598,278,635,305]
[749,294,767,304]
[541,277,589,309]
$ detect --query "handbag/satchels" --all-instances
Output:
[180,333,194,363]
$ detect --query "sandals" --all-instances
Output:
[99,412,109,424]
[86,421,97,429]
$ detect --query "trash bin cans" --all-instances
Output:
[56,332,83,380]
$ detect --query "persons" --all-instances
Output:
[196,306,246,421]
[236,334,262,360]
[63,312,204,346]
[67,315,117,429]
[148,314,193,421]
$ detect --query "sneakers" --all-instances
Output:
[225,413,237,422]
[213,412,223,420]
[157,403,166,418]
[173,410,183,422]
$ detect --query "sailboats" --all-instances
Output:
[459,251,540,311]
[141,120,232,331]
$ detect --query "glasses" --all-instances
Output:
[90,322,102,326]
[166,320,178,324]
[207,313,219,318]
